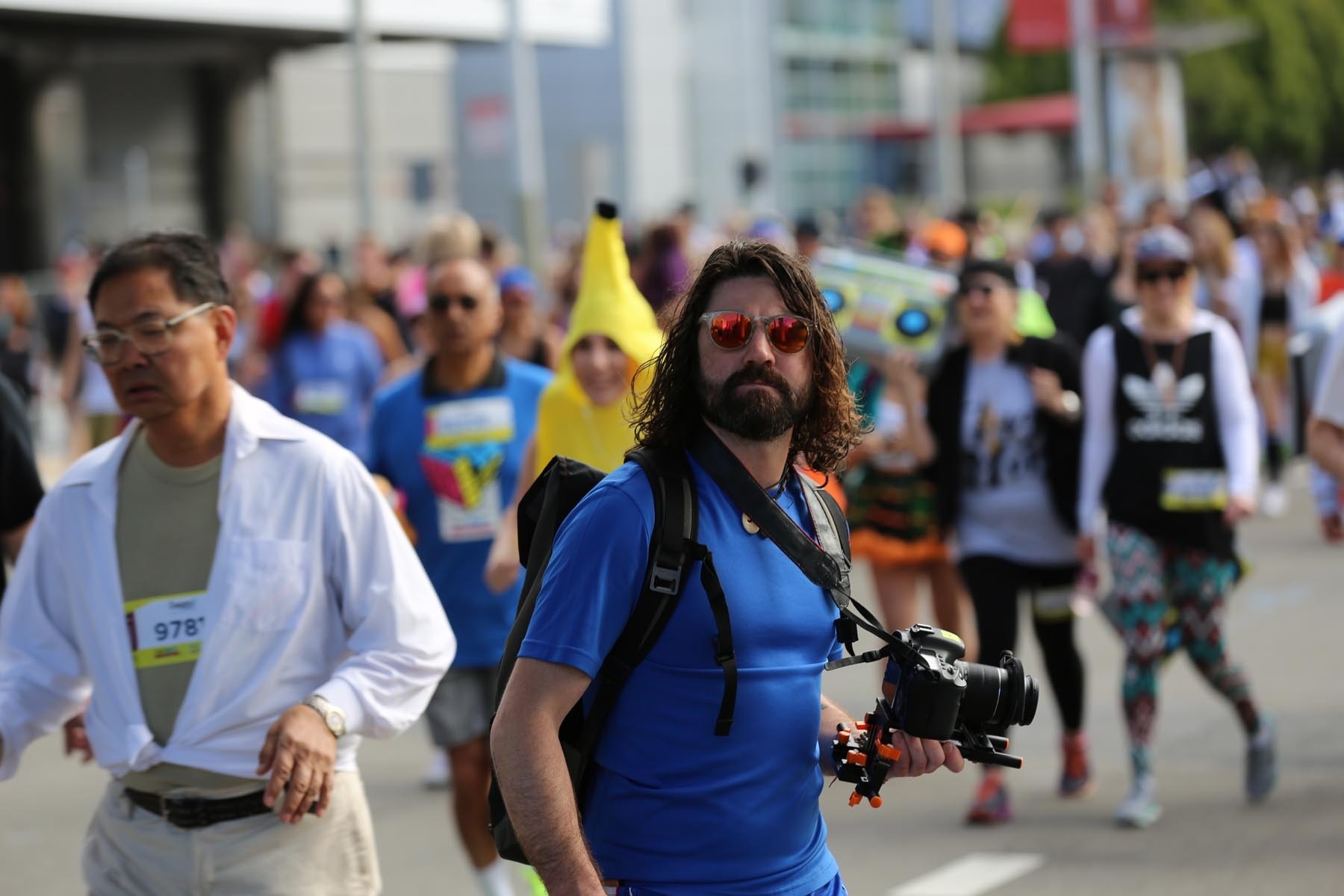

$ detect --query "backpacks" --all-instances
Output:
[482,445,849,868]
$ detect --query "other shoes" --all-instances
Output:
[423,758,453,789]
[966,790,1014,826]
[1117,797,1164,832]
[1244,728,1279,803]
[1058,744,1090,797]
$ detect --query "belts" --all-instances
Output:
[123,785,289,828]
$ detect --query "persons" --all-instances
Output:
[488,242,963,896]
[1,144,1344,894]
[1,231,457,895]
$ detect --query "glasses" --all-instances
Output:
[1137,262,1188,282]
[81,303,218,366]
[698,310,816,356]
[430,294,494,313]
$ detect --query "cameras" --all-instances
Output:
[827,623,1040,809]
